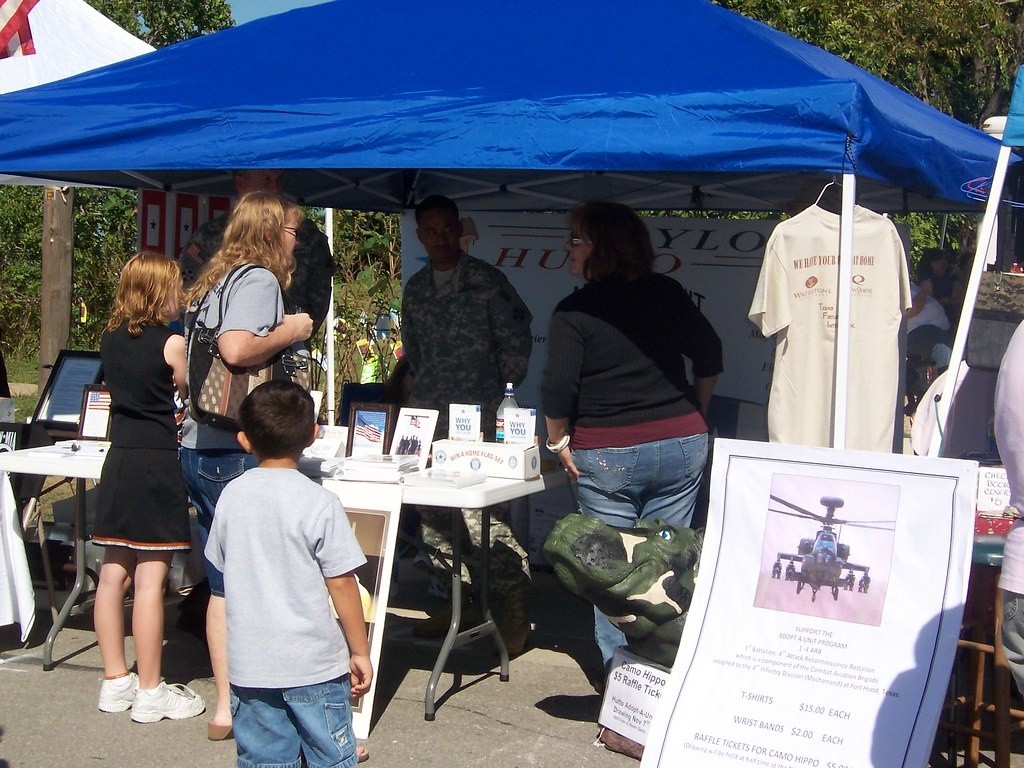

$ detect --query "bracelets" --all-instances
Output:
[545,433,570,453]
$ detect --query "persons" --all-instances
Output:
[542,200,724,694]
[92,250,206,724]
[180,191,371,762]
[402,194,535,656]
[388,356,416,406]
[995,319,1024,693]
[180,169,336,341]
[907,248,976,423]
[204,381,374,767]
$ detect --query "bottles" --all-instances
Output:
[495,383,519,443]
[918,354,937,409]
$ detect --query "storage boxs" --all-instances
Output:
[431,439,541,480]
[25,520,106,612]
[168,515,206,592]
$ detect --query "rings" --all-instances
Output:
[564,468,568,472]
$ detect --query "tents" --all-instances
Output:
[0,1,1023,457]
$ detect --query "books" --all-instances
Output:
[54,439,485,490]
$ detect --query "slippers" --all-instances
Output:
[354,747,369,763]
[208,718,235,741]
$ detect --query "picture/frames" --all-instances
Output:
[30,350,104,432]
[345,400,396,457]
[75,383,112,441]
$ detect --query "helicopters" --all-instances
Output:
[757,483,898,608]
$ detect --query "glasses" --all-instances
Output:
[569,233,596,247]
[281,225,303,242]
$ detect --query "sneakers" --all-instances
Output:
[98,672,140,713]
[130,679,206,724]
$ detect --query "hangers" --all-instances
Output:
[959,175,1024,208]
[815,173,856,207]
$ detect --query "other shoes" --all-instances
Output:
[413,551,434,574]
[903,402,917,413]
[491,607,531,656]
[411,598,450,637]
[428,571,451,600]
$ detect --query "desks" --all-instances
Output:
[0,439,570,722]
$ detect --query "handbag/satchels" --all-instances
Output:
[187,264,312,432]
